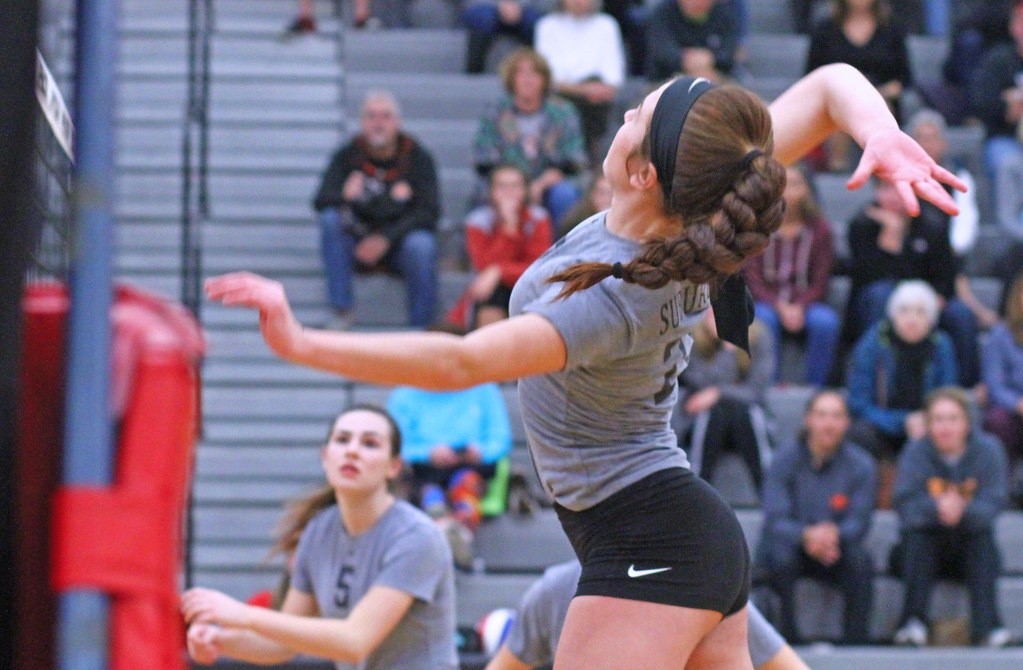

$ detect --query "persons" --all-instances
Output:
[295,0,1023,670]
[886,382,1009,647]
[181,404,462,670]
[315,85,442,329]
[532,0,628,155]
[465,47,588,228]
[741,164,845,389]
[193,59,967,670]
[750,383,882,644]
[804,1,911,132]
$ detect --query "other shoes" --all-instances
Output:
[441,521,476,568]
[981,628,1012,648]
[895,616,927,645]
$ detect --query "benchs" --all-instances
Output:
[346,0,1023,670]
